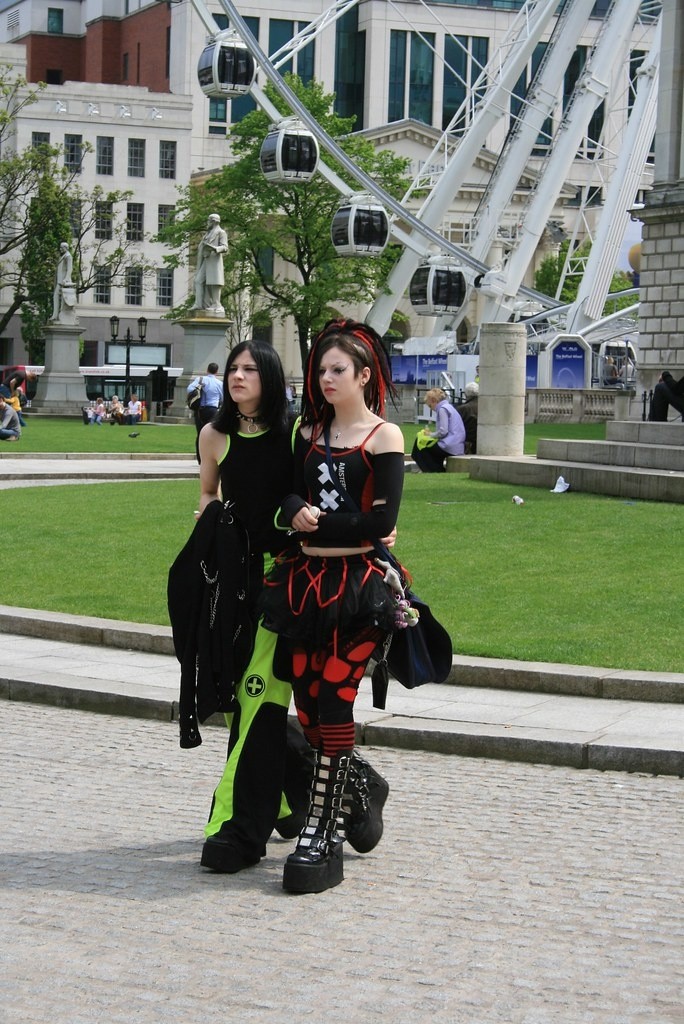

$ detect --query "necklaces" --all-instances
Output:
[335,433,342,439]
[237,410,261,433]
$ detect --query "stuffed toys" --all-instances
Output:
[394,600,420,629]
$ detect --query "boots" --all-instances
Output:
[282,749,354,891]
[345,750,389,853]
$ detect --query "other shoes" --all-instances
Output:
[6,436,19,442]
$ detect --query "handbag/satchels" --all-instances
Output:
[188,376,203,410]
[416,428,438,451]
[372,587,453,710]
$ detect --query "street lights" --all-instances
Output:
[107,312,149,406]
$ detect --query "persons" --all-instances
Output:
[259,320,405,892]
[186,362,293,464]
[197,338,396,872]
[0,372,37,441]
[455,382,479,454]
[410,387,466,474]
[648,371,684,422]
[91,394,142,427]
[603,356,634,377]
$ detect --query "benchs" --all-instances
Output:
[81,406,117,425]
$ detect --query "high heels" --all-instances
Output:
[276,803,310,839]
[200,836,260,872]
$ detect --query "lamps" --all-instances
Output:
[88,103,98,116]
[120,106,130,118]
[57,101,66,114]
[152,108,162,121]
